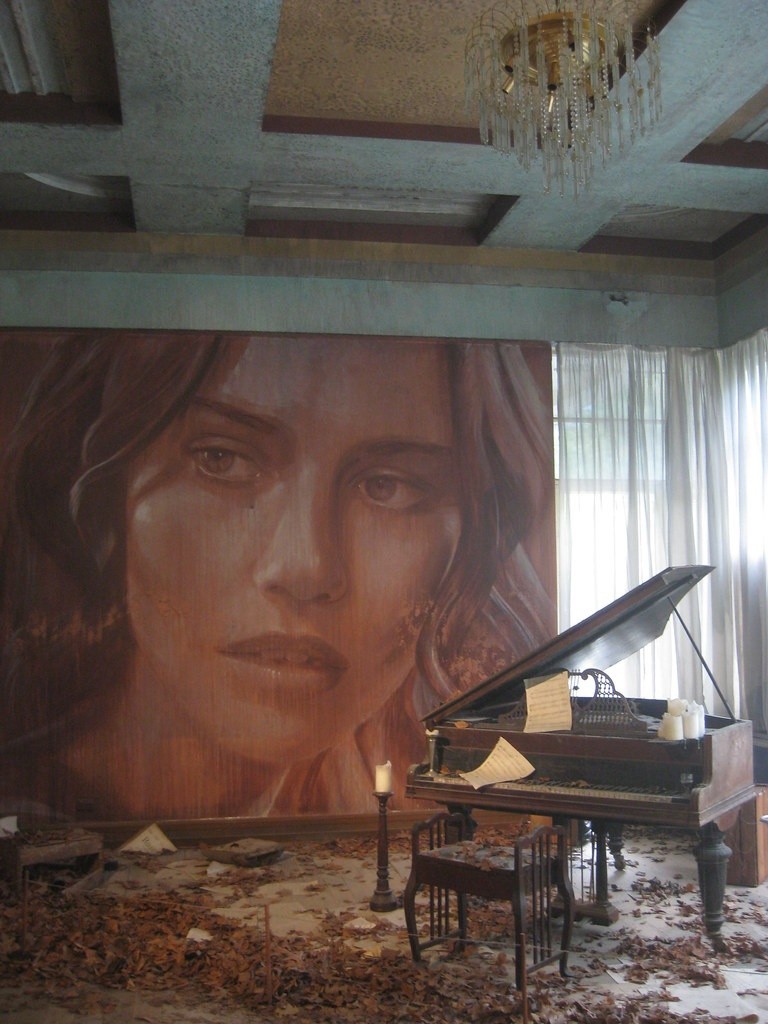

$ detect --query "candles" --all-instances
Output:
[657,698,705,741]
[375,760,392,792]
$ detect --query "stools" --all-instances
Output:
[404,812,575,991]
[0,828,104,956]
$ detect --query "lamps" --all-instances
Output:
[465,0,663,198]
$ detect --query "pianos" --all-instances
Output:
[404,565,759,940]
